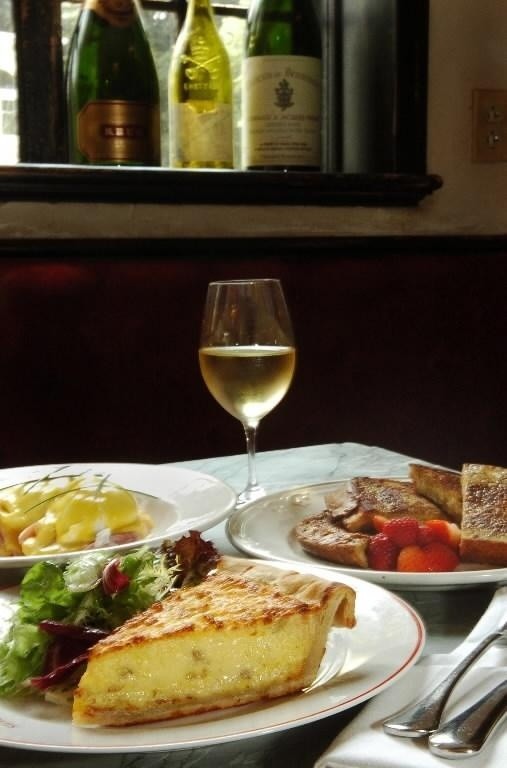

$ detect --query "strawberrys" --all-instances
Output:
[368,516,462,574]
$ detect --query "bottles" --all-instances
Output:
[244,2,324,172]
[66,2,161,167]
[168,5,233,170]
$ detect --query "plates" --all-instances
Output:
[224,474,505,592]
[1,461,236,573]
[1,562,428,753]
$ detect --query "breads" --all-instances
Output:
[67,555,357,729]
[295,460,507,566]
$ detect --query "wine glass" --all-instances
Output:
[199,278,297,510]
